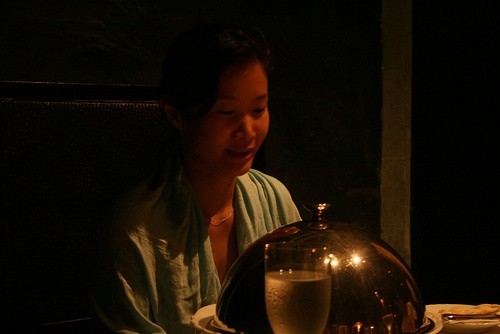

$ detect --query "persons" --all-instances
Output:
[87,18,303,334]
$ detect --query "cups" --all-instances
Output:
[264,242,331,334]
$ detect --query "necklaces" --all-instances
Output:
[200,208,234,223]
